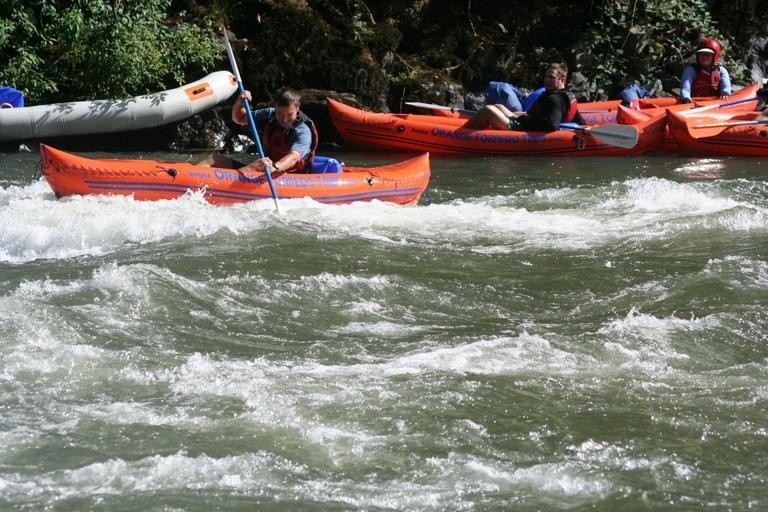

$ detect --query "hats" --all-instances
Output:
[695,48,715,54]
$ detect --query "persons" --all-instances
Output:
[462,60,586,134]
[680,39,732,104]
[232,85,318,175]
[461,69,526,111]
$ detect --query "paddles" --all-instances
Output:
[678,95,760,116]
[406,102,638,149]
[221,22,286,214]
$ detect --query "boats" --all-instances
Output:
[325,94,668,162]
[576,80,768,158]
[0,67,239,152]
[36,141,432,205]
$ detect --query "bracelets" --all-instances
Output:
[272,162,280,174]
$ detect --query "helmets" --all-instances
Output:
[696,39,722,63]
